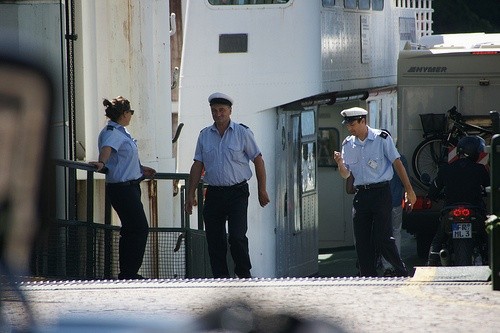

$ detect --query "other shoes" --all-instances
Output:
[398,263,407,276]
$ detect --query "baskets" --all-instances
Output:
[419,113,448,133]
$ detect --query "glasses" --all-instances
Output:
[127,110,134,115]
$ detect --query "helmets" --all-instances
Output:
[457,135,486,156]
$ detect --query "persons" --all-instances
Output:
[185,93,271,278]
[89,97,157,279]
[333,106,417,277]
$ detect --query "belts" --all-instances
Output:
[208,182,247,190]
[358,182,390,189]
[111,178,141,186]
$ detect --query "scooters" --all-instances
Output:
[433,200,483,266]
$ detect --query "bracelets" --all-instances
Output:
[98,160,106,168]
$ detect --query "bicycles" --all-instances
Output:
[411,105,500,187]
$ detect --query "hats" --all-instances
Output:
[208,93,234,107]
[340,107,368,125]
